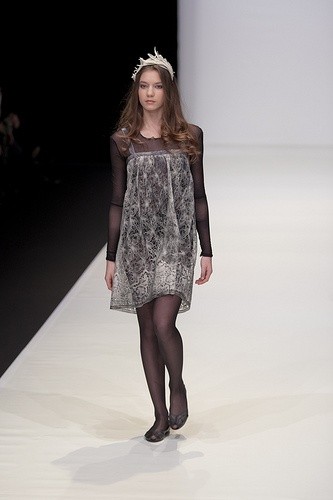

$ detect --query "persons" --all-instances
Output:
[104,45,213,443]
[0,112,21,175]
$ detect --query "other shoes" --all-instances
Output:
[169,407,188,430]
[145,419,170,442]
